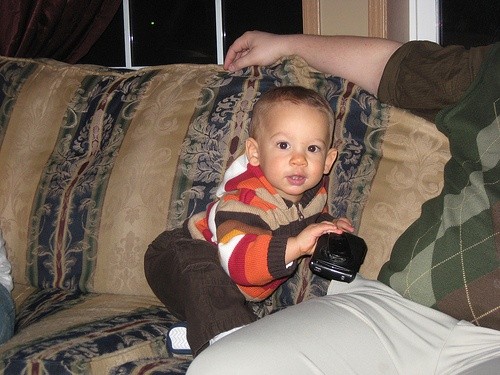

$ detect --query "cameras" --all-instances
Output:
[309,231,367,283]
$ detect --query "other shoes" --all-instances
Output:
[166,321,194,359]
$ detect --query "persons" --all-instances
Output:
[186,30,500,375]
[0,228,16,345]
[144,87,355,360]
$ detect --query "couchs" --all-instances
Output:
[0,56,452,375]
